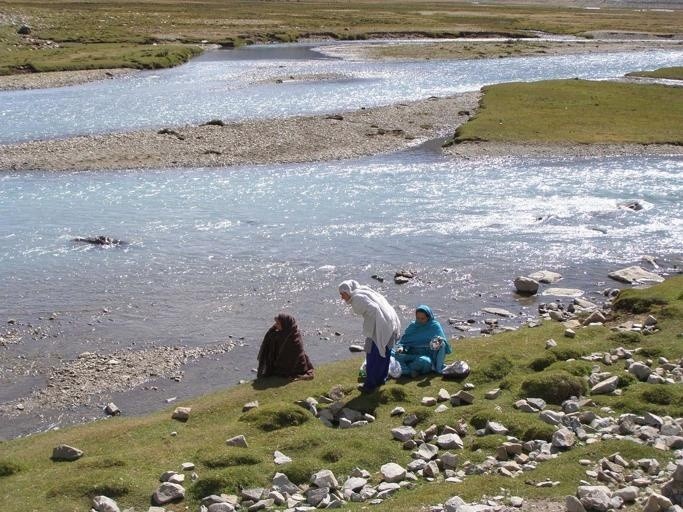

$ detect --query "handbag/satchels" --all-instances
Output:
[440,358,471,379]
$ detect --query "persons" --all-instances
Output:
[256,314,313,380]
[388,304,452,380]
[338,279,400,394]
